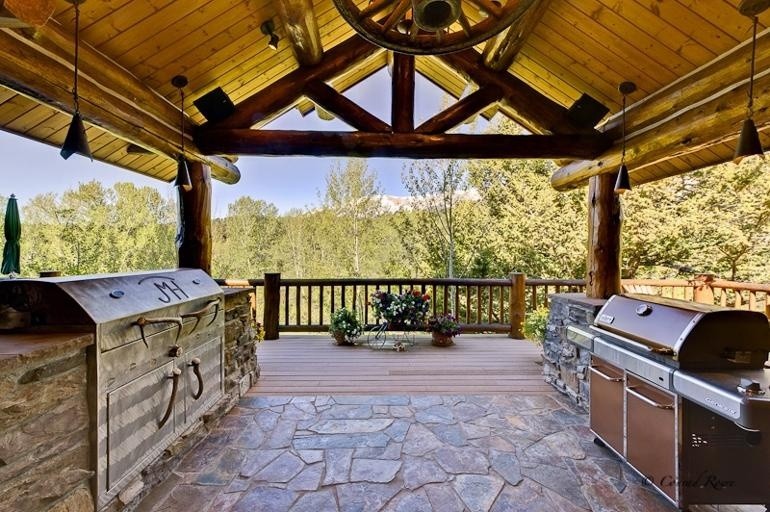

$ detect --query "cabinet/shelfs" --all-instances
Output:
[0,270,227,512]
[588,351,770,512]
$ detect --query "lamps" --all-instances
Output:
[732,0,770,165]
[171,76,192,192]
[611,83,637,194]
[57,0,96,162]
[260,19,279,50]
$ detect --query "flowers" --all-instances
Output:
[424,315,465,338]
[367,288,432,325]
[328,309,364,345]
[518,304,550,347]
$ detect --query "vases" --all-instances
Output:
[331,330,348,345]
[431,330,454,346]
